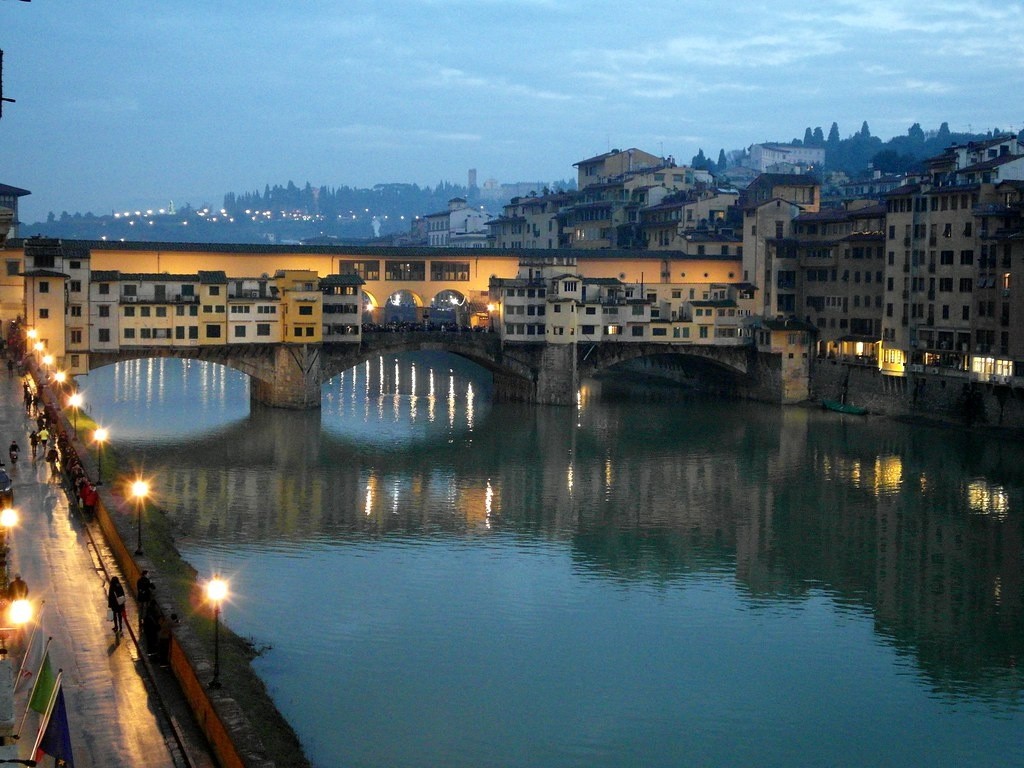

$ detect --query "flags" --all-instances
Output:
[21,603,74,768]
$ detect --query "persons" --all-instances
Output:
[108,576,126,631]
[9,573,29,601]
[7,358,99,523]
[362,321,494,333]
[137,570,156,621]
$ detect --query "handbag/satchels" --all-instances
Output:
[107,608,114,621]
[117,596,125,605]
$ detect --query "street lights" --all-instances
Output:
[69,394,79,442]
[36,343,43,371]
[54,372,65,412]
[28,329,36,356]
[45,356,52,379]
[131,481,147,556]
[94,429,104,486]
[205,579,228,690]
